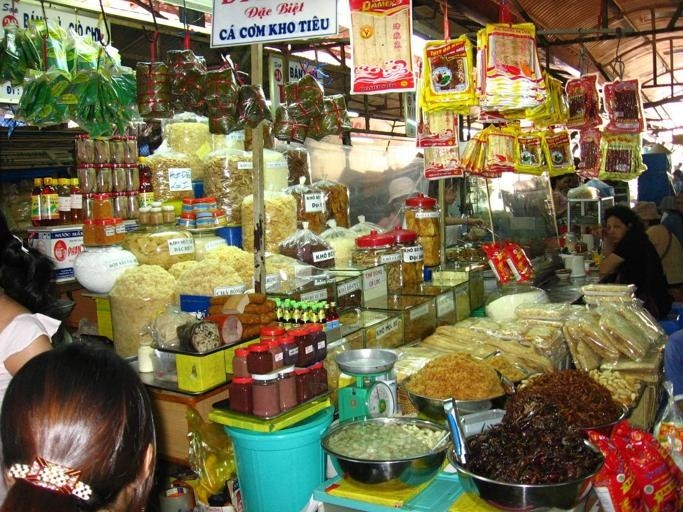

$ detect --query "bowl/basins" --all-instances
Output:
[402,373,516,421]
[553,268,571,279]
[445,427,607,511]
[555,278,571,287]
[316,414,453,494]
[605,398,632,432]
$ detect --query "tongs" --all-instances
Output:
[442,394,469,467]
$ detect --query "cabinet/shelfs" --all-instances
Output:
[568,193,615,233]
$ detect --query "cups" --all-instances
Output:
[565,256,586,278]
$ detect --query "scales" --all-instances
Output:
[334,348,399,422]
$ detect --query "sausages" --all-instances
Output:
[208,294,277,343]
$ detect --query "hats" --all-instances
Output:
[631,200,661,220]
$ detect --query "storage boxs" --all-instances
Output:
[150,335,260,394]
[26,223,84,283]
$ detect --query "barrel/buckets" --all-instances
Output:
[223,401,335,512]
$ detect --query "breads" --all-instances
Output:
[424,284,669,383]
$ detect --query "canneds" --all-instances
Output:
[75,133,228,245]
[229,324,329,419]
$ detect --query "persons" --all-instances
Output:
[0,212,61,509]
[664,329,682,418]
[0,336,162,509]
[548,173,576,221]
[593,164,682,322]
[376,177,423,228]
[428,178,486,245]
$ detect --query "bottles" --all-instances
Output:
[564,232,576,251]
[136,156,155,208]
[159,205,175,223]
[273,297,342,333]
[137,339,154,373]
[139,206,151,224]
[149,201,162,210]
[149,208,162,224]
[29,176,84,226]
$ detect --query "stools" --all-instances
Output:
[658,300,683,335]
